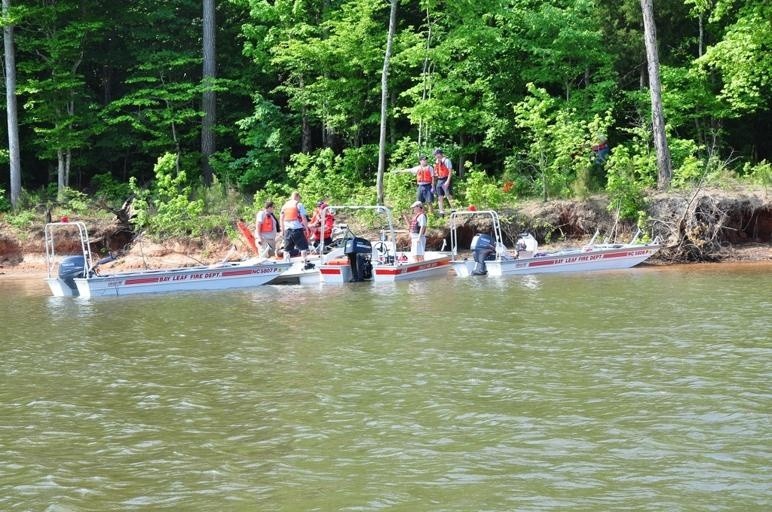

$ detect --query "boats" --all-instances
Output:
[447,208,659,278]
[210,207,458,286]
[44,221,296,298]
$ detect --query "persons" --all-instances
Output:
[433,148,459,212]
[307,200,338,249]
[406,199,428,262]
[387,155,437,209]
[253,199,282,261]
[279,190,316,270]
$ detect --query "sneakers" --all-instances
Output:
[304,263,315,270]
[439,209,457,218]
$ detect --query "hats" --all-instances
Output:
[433,149,442,155]
[410,201,423,208]
[316,201,328,209]
[419,155,428,160]
